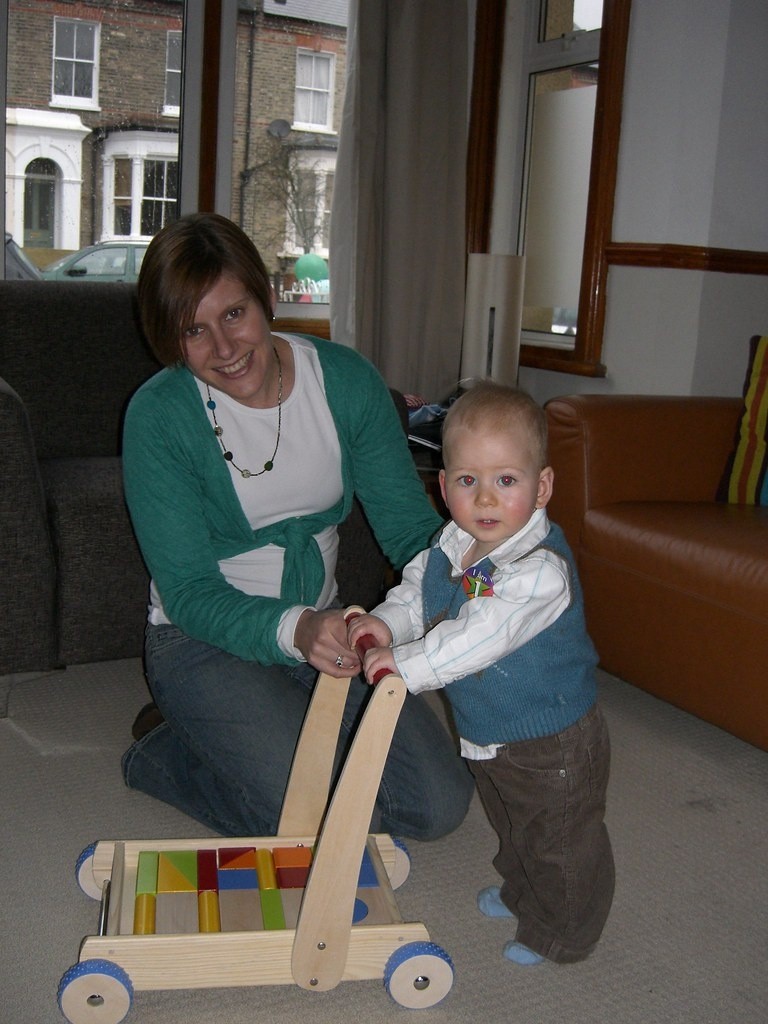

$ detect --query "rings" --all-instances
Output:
[335,656,344,667]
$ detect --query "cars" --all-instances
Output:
[3,233,46,282]
[40,237,156,282]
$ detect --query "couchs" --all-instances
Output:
[534,386,768,754]
[0,278,167,678]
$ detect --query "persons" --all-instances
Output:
[116,211,479,845]
[345,384,616,962]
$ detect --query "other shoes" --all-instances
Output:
[131,701,164,744]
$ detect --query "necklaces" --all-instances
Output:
[204,345,284,479]
